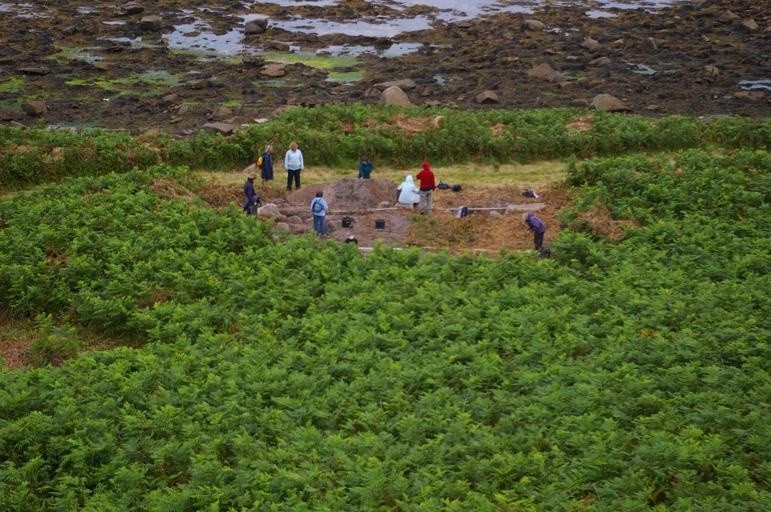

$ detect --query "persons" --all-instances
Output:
[417,162,435,215]
[243,174,258,215]
[358,157,372,179]
[311,191,330,234]
[283,141,304,191]
[396,175,421,204]
[523,212,546,250]
[262,144,274,187]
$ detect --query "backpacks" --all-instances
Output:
[313,202,322,212]
[257,153,269,169]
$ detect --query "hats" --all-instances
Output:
[247,174,256,179]
